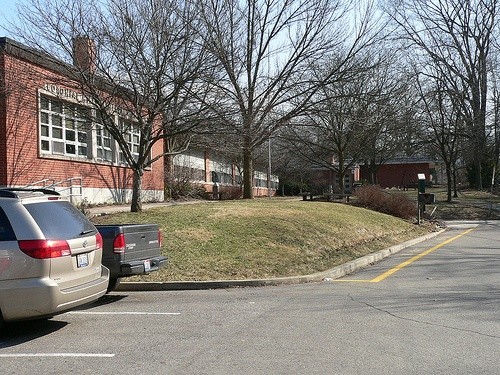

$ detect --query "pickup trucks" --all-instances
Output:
[95,222,169,292]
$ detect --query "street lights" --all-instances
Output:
[268,134,280,198]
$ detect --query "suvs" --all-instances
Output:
[1,185,110,324]
